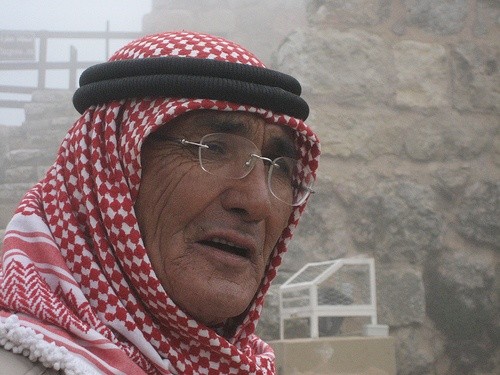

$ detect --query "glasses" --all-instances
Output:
[149,131,317,207]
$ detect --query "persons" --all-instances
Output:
[0,29,323,375]
